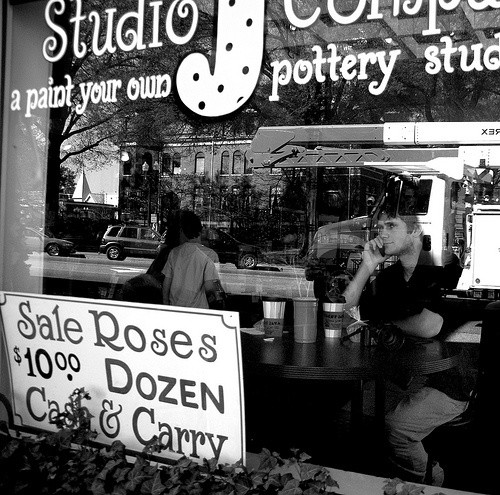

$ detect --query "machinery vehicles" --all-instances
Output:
[246,120,500,303]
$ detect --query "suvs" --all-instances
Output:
[98,223,163,261]
[158,226,265,269]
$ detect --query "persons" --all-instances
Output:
[144,220,186,305]
[341,197,475,487]
[162,214,221,309]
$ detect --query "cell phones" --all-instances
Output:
[379,245,386,257]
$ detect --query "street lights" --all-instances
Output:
[142,161,159,226]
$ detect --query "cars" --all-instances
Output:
[22,224,75,257]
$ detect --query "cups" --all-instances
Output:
[293,297,319,343]
[323,295,346,338]
[263,297,286,337]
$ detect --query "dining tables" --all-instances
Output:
[241,331,467,422]
